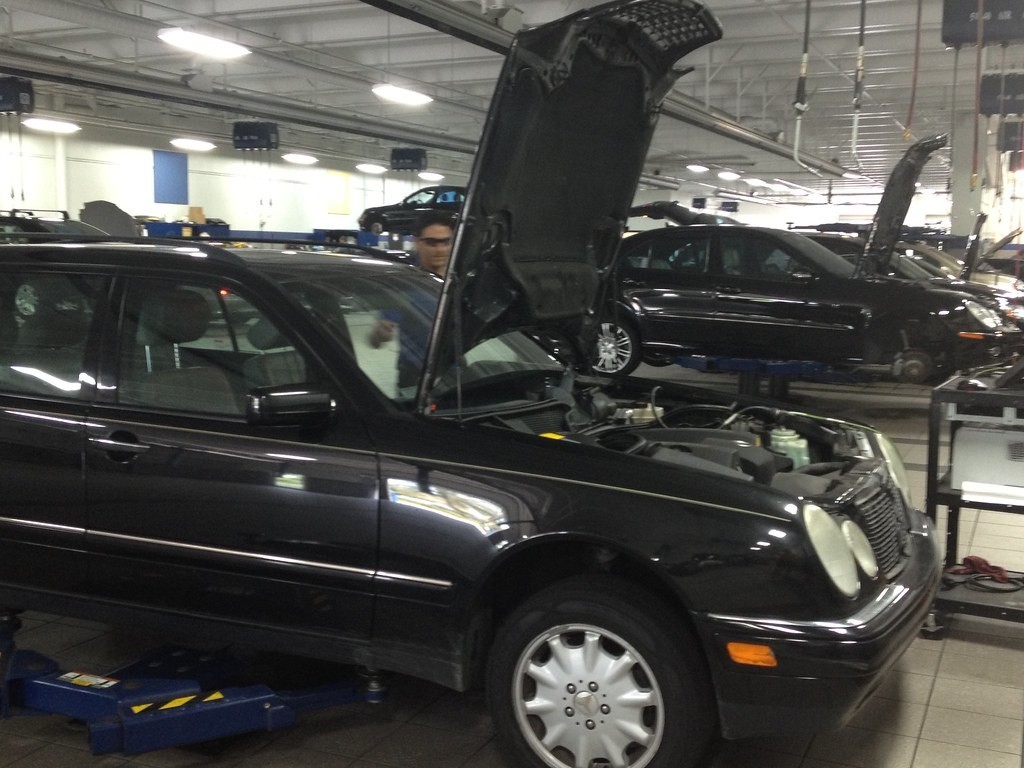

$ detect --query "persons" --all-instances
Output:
[370,209,457,399]
[357,213,389,249]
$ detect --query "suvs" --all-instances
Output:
[0,0,943,768]
[0,209,115,319]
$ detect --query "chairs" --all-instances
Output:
[125,287,242,420]
[0,308,92,397]
[237,314,308,386]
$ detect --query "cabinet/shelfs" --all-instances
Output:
[927,389,1024,623]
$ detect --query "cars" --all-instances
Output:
[585,132,1024,383]
[358,185,466,236]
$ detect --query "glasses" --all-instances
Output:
[418,236,452,247]
